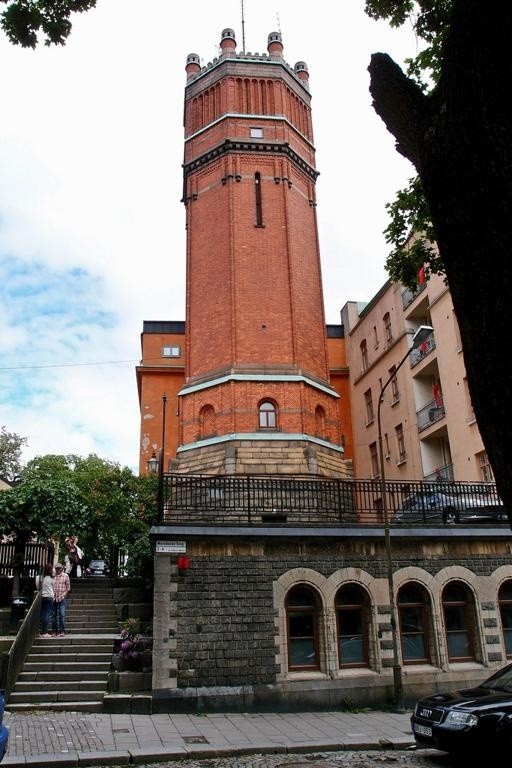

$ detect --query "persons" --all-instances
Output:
[51,563,71,636]
[60,538,76,576]
[73,537,85,577]
[40,565,55,638]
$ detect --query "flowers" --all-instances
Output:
[117,615,151,659]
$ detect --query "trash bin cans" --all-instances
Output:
[10,596,28,628]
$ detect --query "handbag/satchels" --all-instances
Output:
[68,552,80,564]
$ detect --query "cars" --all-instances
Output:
[391,488,509,523]
[411,663,512,765]
[84,559,110,577]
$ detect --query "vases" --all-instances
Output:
[125,657,144,671]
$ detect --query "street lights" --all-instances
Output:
[377,323,436,714]
[147,454,159,473]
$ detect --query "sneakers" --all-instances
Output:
[52,632,57,637]
[39,633,43,638]
[60,631,64,636]
[43,633,52,638]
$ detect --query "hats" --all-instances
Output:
[55,563,63,569]
